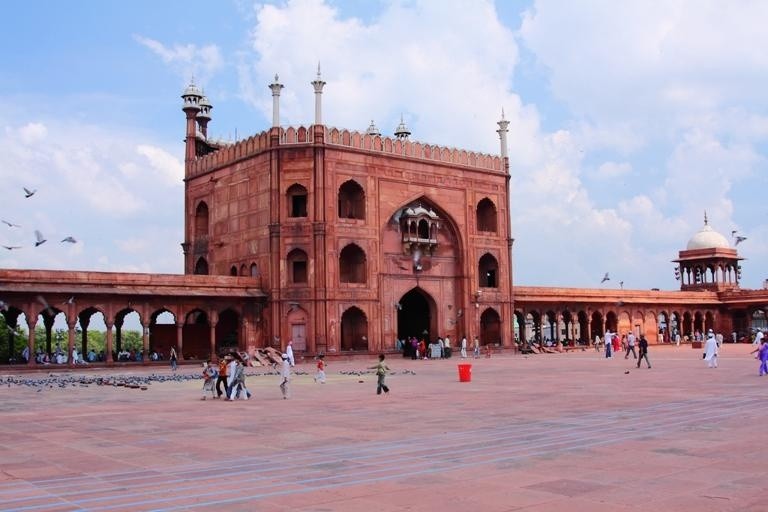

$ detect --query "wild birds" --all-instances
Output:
[1,218,23,229]
[35,230,47,247]
[1,244,23,251]
[23,187,37,199]
[60,235,77,244]
[731,230,747,246]
[600,271,611,284]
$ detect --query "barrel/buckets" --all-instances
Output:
[458,363,471,381]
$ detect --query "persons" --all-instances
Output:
[286,341,295,367]
[659,327,768,376]
[515,329,651,370]
[397,334,493,359]
[313,355,328,384]
[22,343,251,401]
[279,354,290,399]
[366,354,390,395]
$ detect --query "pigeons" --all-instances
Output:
[0,373,203,390]
